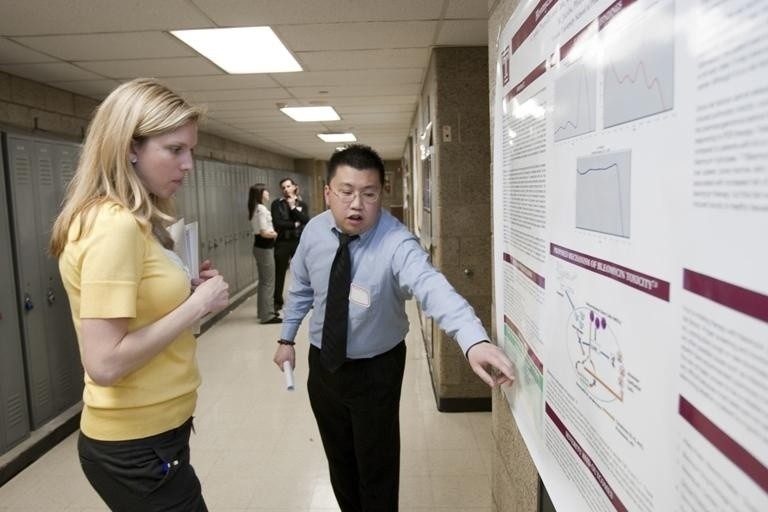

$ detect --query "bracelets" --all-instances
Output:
[277,340,295,346]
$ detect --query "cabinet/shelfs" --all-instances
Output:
[1,139,307,450]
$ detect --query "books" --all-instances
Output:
[164,217,200,335]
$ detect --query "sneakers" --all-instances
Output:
[262,312,283,324]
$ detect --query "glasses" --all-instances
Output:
[328,184,379,202]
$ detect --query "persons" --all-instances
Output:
[51,78,230,511]
[271,142,517,511]
[248,183,283,324]
[270,178,309,311]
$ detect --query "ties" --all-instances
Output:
[319,231,361,374]
